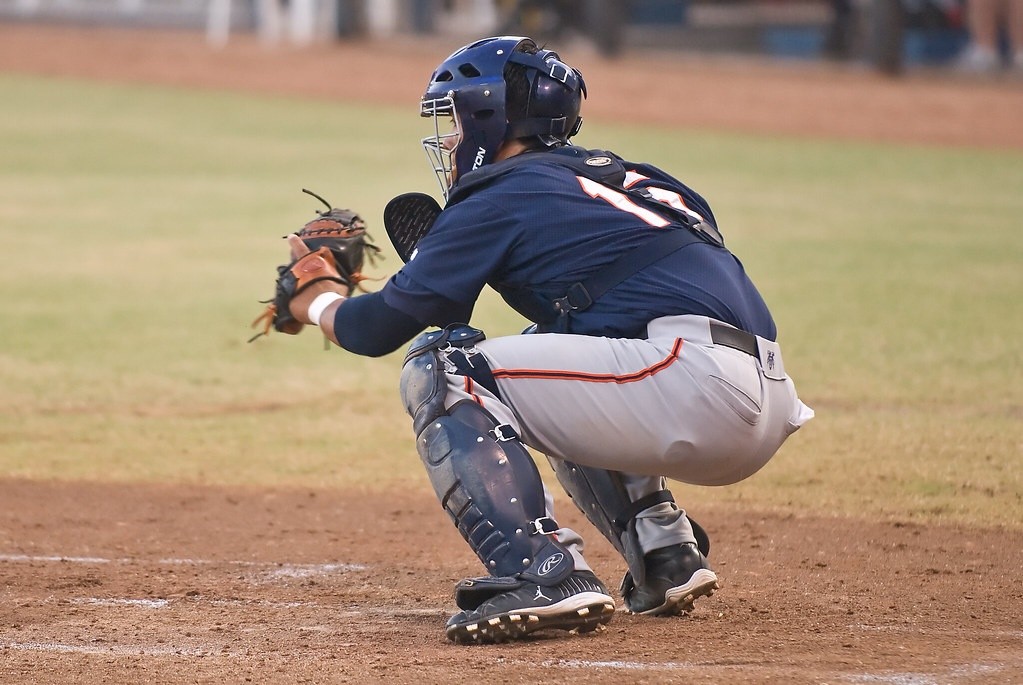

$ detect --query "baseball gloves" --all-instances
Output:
[265,207,369,336]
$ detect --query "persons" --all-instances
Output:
[250,34,814,642]
[961,0,1023,71]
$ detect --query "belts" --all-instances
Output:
[637,324,760,359]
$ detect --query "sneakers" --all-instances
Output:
[616,543,720,616]
[444,574,615,646]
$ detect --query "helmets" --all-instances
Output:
[420,35,587,203]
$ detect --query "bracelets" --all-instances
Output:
[307,291,345,325]
